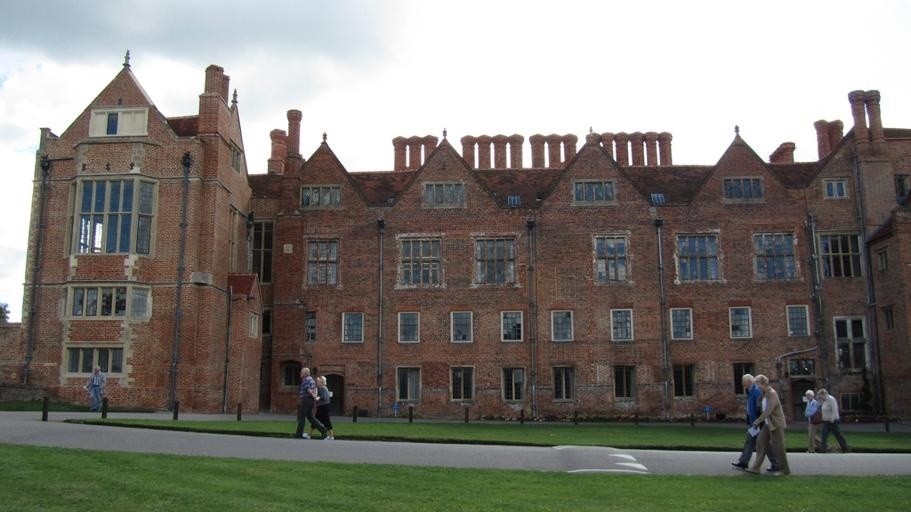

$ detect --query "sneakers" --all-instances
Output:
[324,436,335,441]
[319,428,329,440]
[302,433,312,439]
[806,444,850,453]
[292,433,303,438]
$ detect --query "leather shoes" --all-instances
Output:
[767,464,778,471]
[773,471,786,476]
[731,460,748,469]
[744,467,761,475]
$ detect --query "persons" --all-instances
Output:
[293,365,328,441]
[803,388,829,454]
[301,375,336,441]
[744,373,790,476]
[813,387,853,453]
[83,364,108,412]
[730,372,782,471]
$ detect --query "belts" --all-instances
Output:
[91,384,101,387]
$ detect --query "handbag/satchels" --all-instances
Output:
[810,411,823,425]
